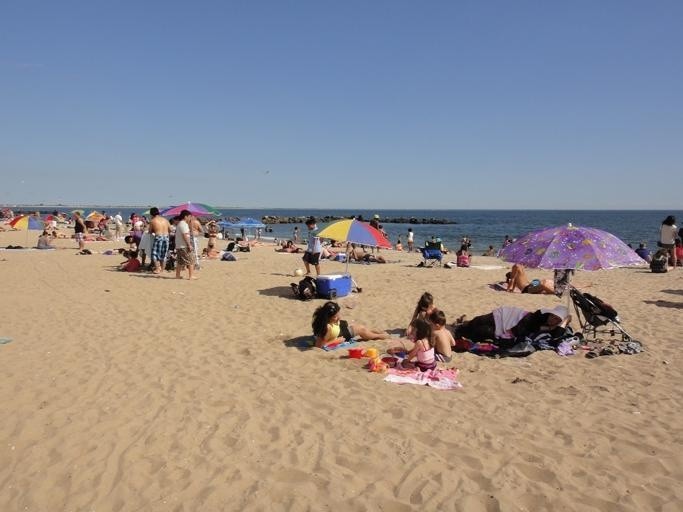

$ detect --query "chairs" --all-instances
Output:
[420,240,442,266]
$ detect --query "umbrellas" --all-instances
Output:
[498,223,650,311]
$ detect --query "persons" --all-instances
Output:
[274,213,521,274]
[313,301,389,349]
[401,292,435,341]
[505,264,553,297]
[450,305,572,342]
[1,202,273,280]
[625,214,683,275]
[428,308,456,364]
[397,318,438,371]
[502,271,554,288]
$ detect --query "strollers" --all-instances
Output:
[572,285,631,342]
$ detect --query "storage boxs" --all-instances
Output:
[317,274,351,298]
